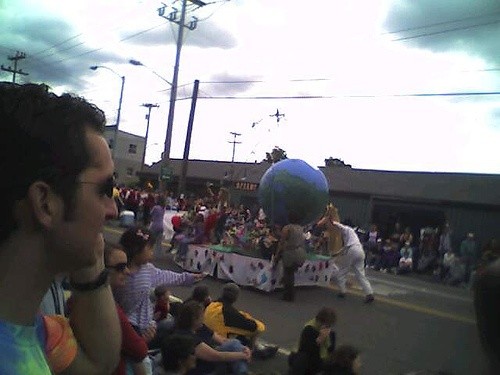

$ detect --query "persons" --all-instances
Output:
[0,80,123,375]
[102,181,500,375]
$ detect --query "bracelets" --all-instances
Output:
[62,268,110,291]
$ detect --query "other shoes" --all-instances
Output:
[165,248,172,253]
[258,344,278,358]
[364,295,374,303]
[175,255,186,261]
[337,292,345,298]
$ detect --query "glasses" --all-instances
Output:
[75,170,118,198]
[105,262,128,272]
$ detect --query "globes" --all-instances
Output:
[257,156,329,239]
